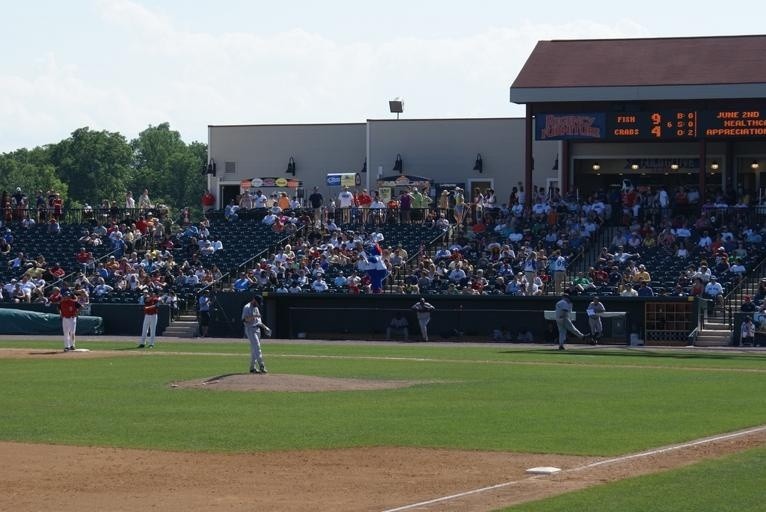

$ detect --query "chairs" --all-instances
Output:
[1,208,766,311]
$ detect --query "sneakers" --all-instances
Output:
[64,347,69,352]
[559,346,566,351]
[260,366,267,373]
[70,345,76,351]
[582,333,591,341]
[149,344,154,349]
[136,343,144,349]
[250,368,261,374]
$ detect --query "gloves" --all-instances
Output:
[264,328,271,336]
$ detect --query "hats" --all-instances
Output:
[454,187,461,191]
[718,246,725,250]
[255,294,264,306]
[440,189,450,196]
[16,187,21,192]
[342,185,350,190]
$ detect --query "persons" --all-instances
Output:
[240,296,272,374]
[1,177,764,352]
